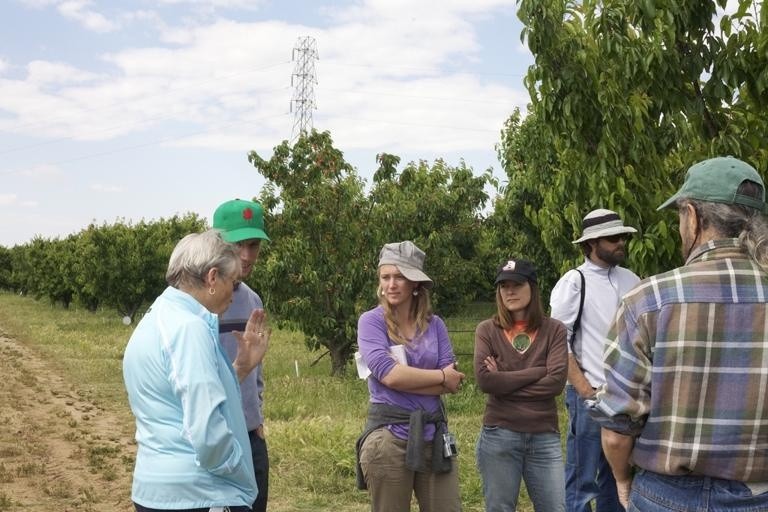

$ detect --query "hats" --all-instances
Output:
[656,155,766,212]
[570,209,638,244]
[494,259,537,285]
[213,198,271,243]
[378,240,434,289]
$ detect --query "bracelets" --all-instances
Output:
[440,369,445,385]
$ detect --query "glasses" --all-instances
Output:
[601,233,628,241]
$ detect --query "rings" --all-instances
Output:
[258,332,265,338]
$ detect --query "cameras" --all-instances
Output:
[444,437,458,457]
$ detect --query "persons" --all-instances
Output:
[213,200,272,512]
[121,230,272,512]
[356,240,467,512]
[584,156,768,512]
[550,208,643,512]
[471,259,569,511]
